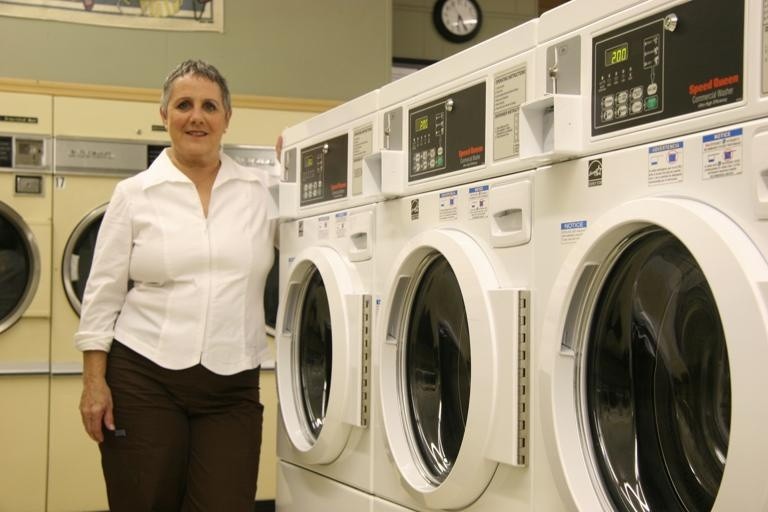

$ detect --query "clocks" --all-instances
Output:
[433,1,481,42]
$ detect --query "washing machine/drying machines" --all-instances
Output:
[0,0,767,512]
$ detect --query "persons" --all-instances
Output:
[77,57,285,512]
